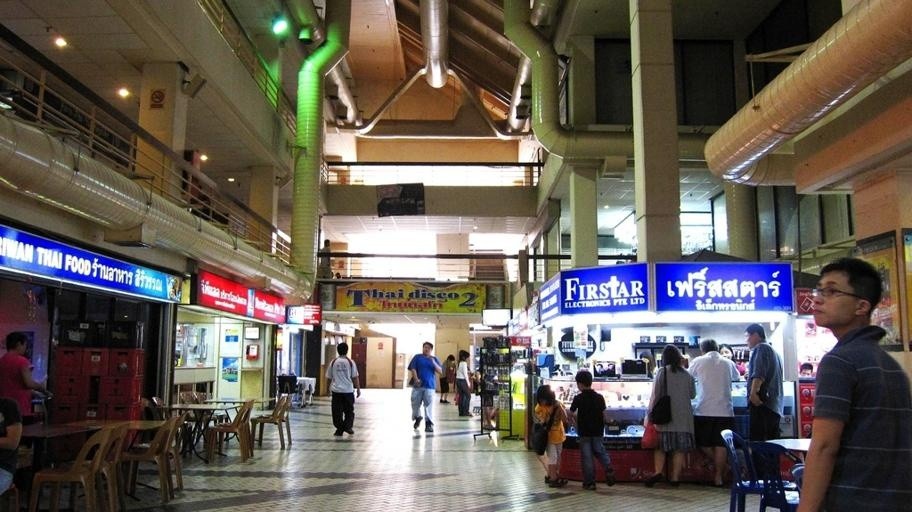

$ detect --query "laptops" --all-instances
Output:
[591,360,616,381]
[620,359,651,380]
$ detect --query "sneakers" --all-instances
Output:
[334,427,343,436]
[544,478,569,489]
[443,400,449,403]
[342,426,354,434]
[459,412,472,416]
[414,416,423,429]
[582,478,597,490]
[605,465,617,487]
[425,422,433,432]
[440,399,444,403]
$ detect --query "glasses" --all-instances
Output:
[812,286,868,302]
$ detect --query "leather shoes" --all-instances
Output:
[644,473,664,488]
[667,481,682,488]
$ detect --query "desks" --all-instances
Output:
[765,439,809,461]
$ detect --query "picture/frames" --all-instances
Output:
[901,227,912,352]
[856,230,904,352]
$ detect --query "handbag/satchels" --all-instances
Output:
[649,394,672,425]
[528,422,549,456]
[640,418,659,449]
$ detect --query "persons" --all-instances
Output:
[0,332,56,424]
[325,342,361,436]
[643,344,696,488]
[687,339,741,486]
[720,344,746,376]
[319,240,332,280]
[439,350,495,429]
[745,324,785,480]
[0,398,23,496]
[407,342,442,432]
[795,257,912,512]
[187,176,210,219]
[569,371,615,490]
[534,385,569,487]
[799,363,813,377]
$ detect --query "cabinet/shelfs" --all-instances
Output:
[473,346,519,443]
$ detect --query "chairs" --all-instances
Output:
[743,440,803,512]
[791,463,805,496]
[0,391,293,512]
[720,429,790,512]
